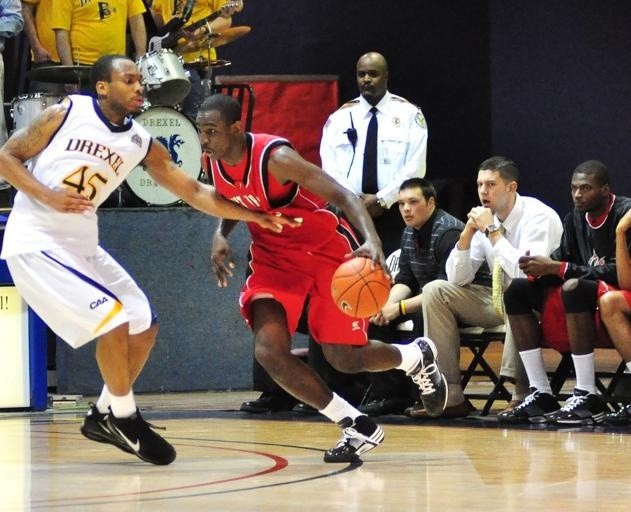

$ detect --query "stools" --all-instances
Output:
[349,317,631,430]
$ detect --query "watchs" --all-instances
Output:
[485,224,498,238]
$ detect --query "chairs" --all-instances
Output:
[205,81,255,135]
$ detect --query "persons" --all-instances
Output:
[498,161,631,424]
[357,178,491,419]
[598,207,631,424]
[0,53,303,467]
[409,153,563,420]
[241,204,361,416]
[0,0,243,207]
[195,93,449,464]
[318,50,430,280]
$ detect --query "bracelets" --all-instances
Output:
[399,300,407,316]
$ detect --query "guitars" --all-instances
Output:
[148,0,246,51]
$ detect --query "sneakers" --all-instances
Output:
[545,386,607,425]
[603,404,631,425]
[405,336,449,418]
[241,391,471,418]
[106,405,176,466]
[323,415,385,462]
[80,402,135,455]
[497,385,561,424]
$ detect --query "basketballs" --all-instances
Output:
[331,257,391,318]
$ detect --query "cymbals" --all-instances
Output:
[186,59,232,68]
[25,66,94,84]
[185,25,252,49]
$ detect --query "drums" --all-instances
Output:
[8,92,62,136]
[135,47,192,104]
[124,104,206,207]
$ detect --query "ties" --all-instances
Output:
[362,107,378,194]
[492,224,505,324]
